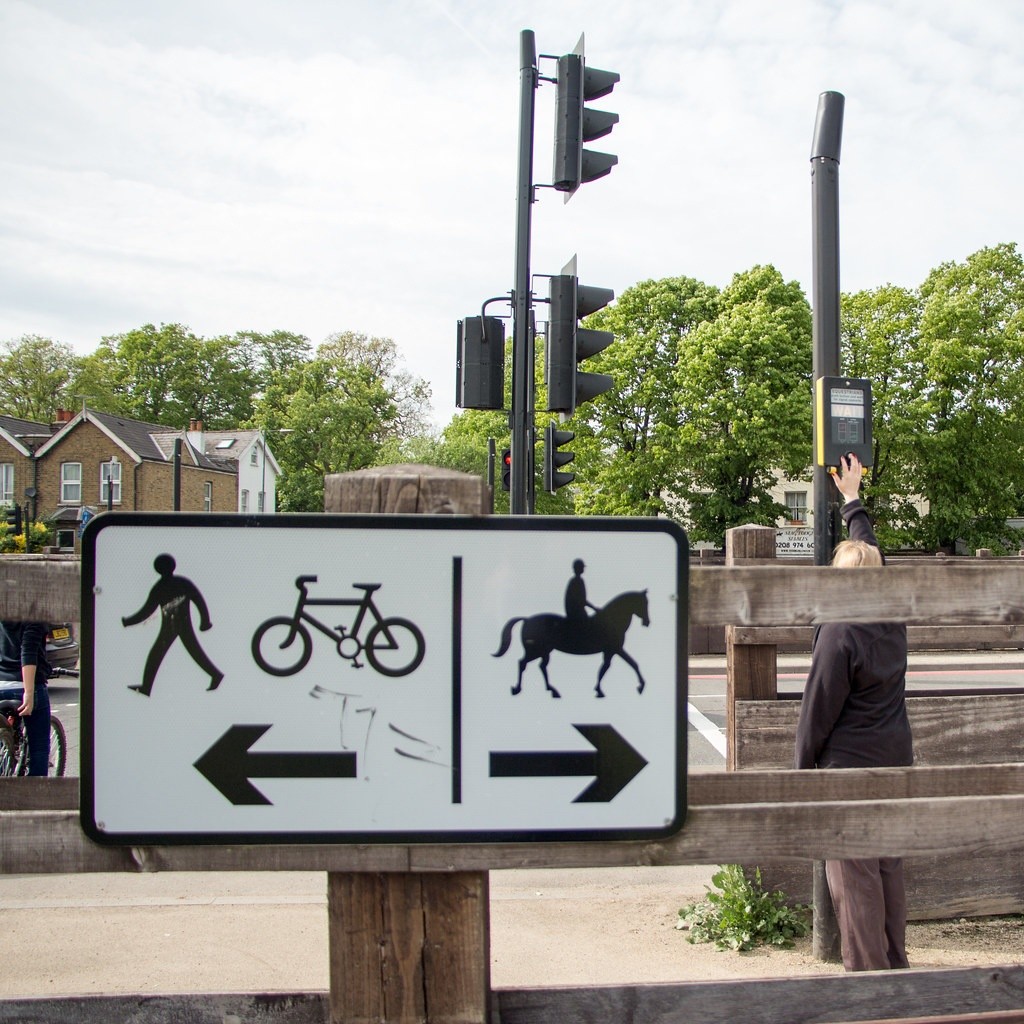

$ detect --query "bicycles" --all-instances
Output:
[0,665,79,777]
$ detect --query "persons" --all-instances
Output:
[794,452,911,973]
[0,622,51,777]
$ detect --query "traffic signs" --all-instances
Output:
[77,510,692,845]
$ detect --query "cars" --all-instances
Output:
[45,621,80,669]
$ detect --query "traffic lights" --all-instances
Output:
[552,30,621,204]
[546,252,615,419]
[6,506,22,536]
[501,449,510,490]
[543,422,576,496]
